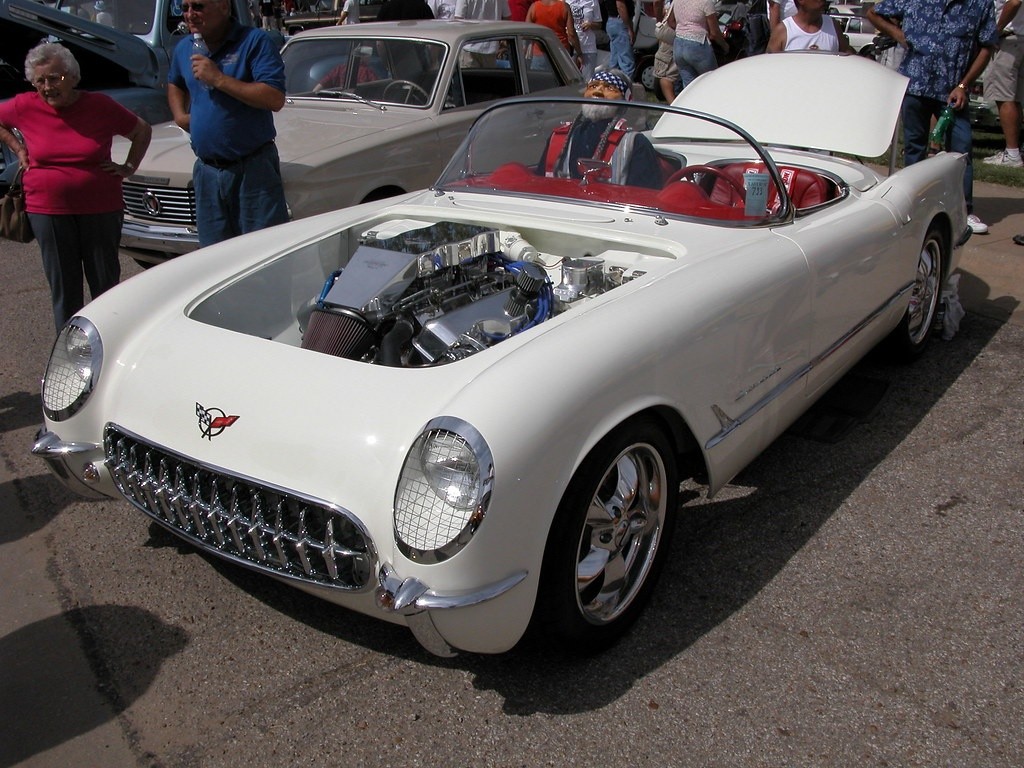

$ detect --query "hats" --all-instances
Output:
[94,1,106,11]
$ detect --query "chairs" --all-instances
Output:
[709,162,826,210]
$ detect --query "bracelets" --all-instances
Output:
[958,84,969,92]
[577,52,582,56]
[126,162,133,168]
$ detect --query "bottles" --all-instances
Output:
[929,103,955,144]
[192,32,216,90]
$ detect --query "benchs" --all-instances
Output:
[418,68,557,100]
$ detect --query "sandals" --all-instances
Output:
[927,147,943,157]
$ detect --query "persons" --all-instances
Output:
[537,68,661,190]
[653,0,799,105]
[867,0,998,234]
[602,0,635,78]
[765,0,854,54]
[249,0,310,51]
[168,0,290,248]
[313,52,380,91]
[377,0,460,70]
[982,0,1024,167]
[335,0,360,25]
[502,0,538,60]
[0,44,153,332]
[562,0,602,81]
[77,0,112,27]
[454,0,511,69]
[524,0,584,73]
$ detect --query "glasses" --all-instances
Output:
[179,1,222,12]
[31,72,72,88]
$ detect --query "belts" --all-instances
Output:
[199,138,274,169]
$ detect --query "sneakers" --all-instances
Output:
[967,214,988,234]
[983,150,1023,168]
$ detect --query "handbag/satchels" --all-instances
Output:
[655,22,676,44]
[0,165,35,243]
